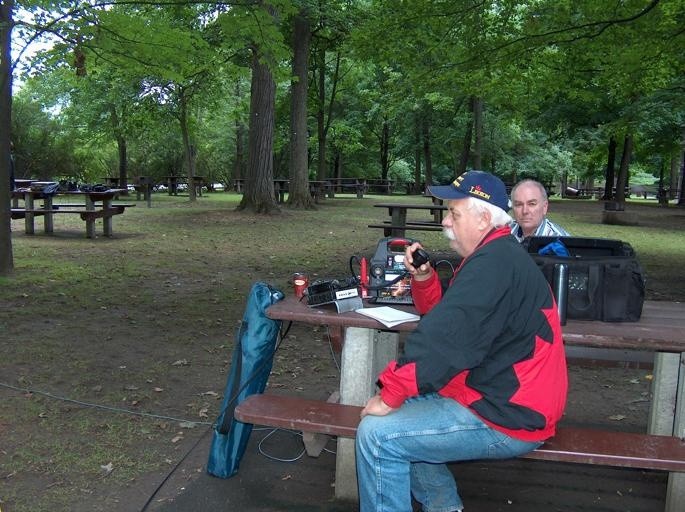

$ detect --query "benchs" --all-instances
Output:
[322,328,657,371]
[234,393,685,511]
[9,202,137,220]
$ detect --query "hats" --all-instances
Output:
[426,168,512,214]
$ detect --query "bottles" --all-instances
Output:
[552,263,571,326]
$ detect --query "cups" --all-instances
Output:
[293,274,309,299]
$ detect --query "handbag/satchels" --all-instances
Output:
[520,235,646,323]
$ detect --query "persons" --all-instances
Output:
[354,169,569,511]
[504,177,568,243]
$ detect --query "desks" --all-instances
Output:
[262,290,684,504]
[12,187,126,239]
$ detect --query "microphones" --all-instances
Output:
[406,249,430,269]
